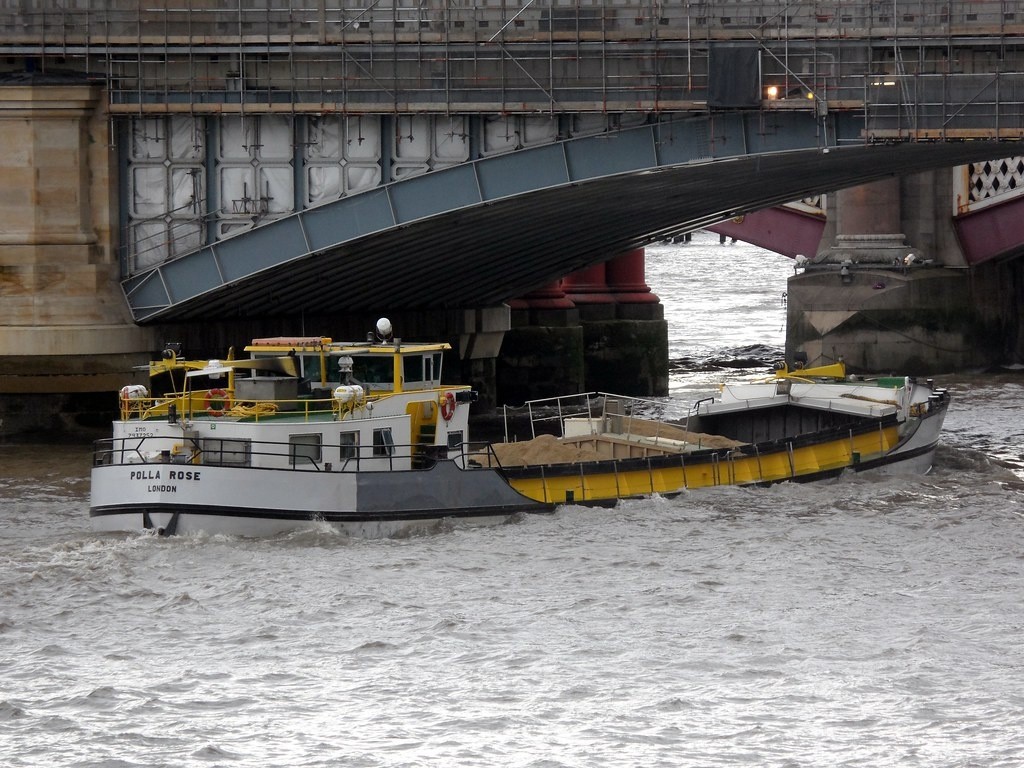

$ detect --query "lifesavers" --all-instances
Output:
[441,393,457,419]
[204,389,229,416]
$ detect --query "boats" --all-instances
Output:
[88,318,952,537]
